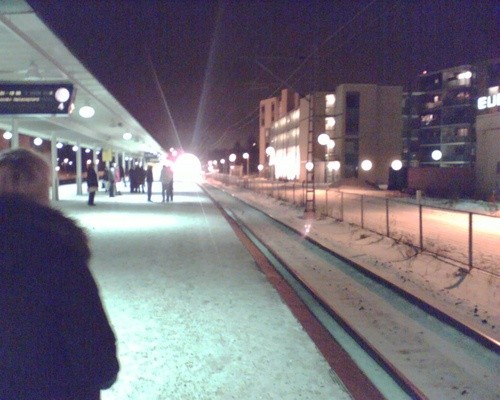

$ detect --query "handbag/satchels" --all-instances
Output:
[88,186,98,193]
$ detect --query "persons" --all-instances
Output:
[0,148,122,400]
[86,162,174,207]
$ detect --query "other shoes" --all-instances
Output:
[88,204,96,206]
